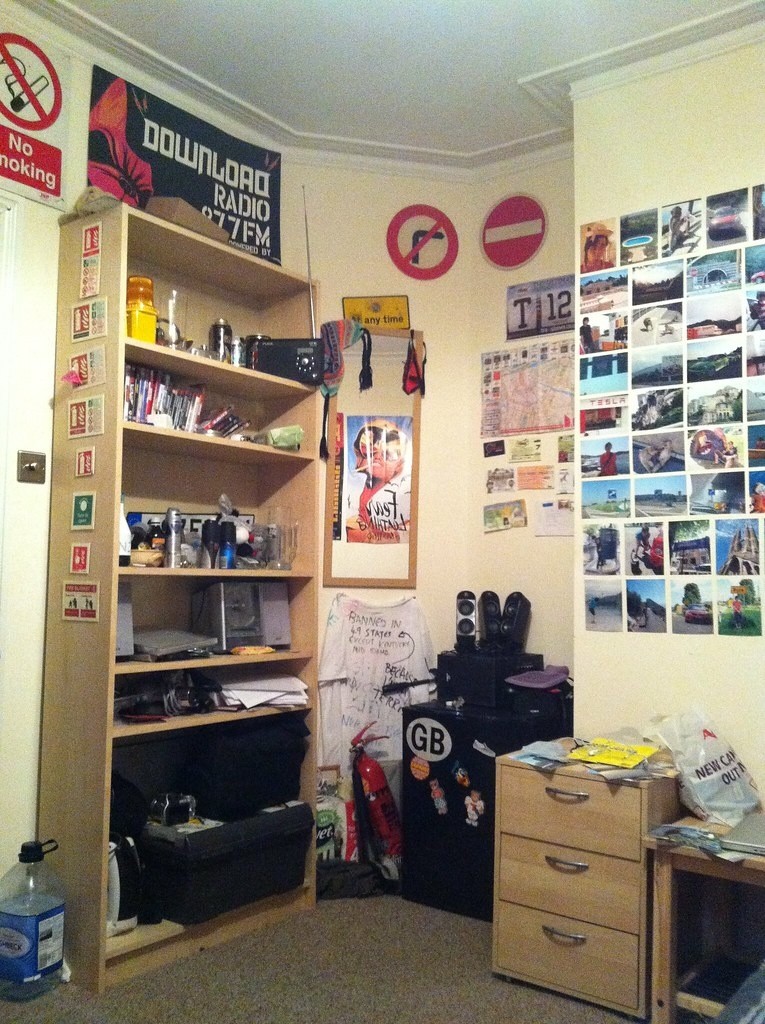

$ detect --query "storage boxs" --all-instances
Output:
[139,800,314,924]
[147,197,230,245]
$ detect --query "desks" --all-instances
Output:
[642,815,765,1024]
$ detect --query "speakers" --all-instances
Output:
[191,582,291,654]
[456,592,531,654]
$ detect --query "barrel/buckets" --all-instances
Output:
[127,304,159,344]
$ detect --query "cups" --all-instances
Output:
[161,290,186,351]
[267,524,297,570]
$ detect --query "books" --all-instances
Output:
[123,363,252,439]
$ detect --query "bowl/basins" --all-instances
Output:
[131,550,162,566]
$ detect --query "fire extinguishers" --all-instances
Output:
[348,721,405,857]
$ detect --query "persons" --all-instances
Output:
[713,440,737,469]
[598,441,619,477]
[577,316,597,353]
[587,596,598,624]
[749,291,764,329]
[591,535,604,569]
[632,525,650,563]
[669,206,684,255]
[731,596,743,629]
[581,225,614,274]
[644,316,674,336]
[640,439,674,474]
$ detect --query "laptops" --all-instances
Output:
[719,812,765,856]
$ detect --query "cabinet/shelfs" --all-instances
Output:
[36,202,322,995]
[492,737,679,1017]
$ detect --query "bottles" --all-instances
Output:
[0,840,67,1002]
[210,318,232,364]
[119,492,132,567]
[147,518,165,550]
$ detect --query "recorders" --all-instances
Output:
[246,338,325,386]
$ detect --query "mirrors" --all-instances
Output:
[323,321,424,589]
